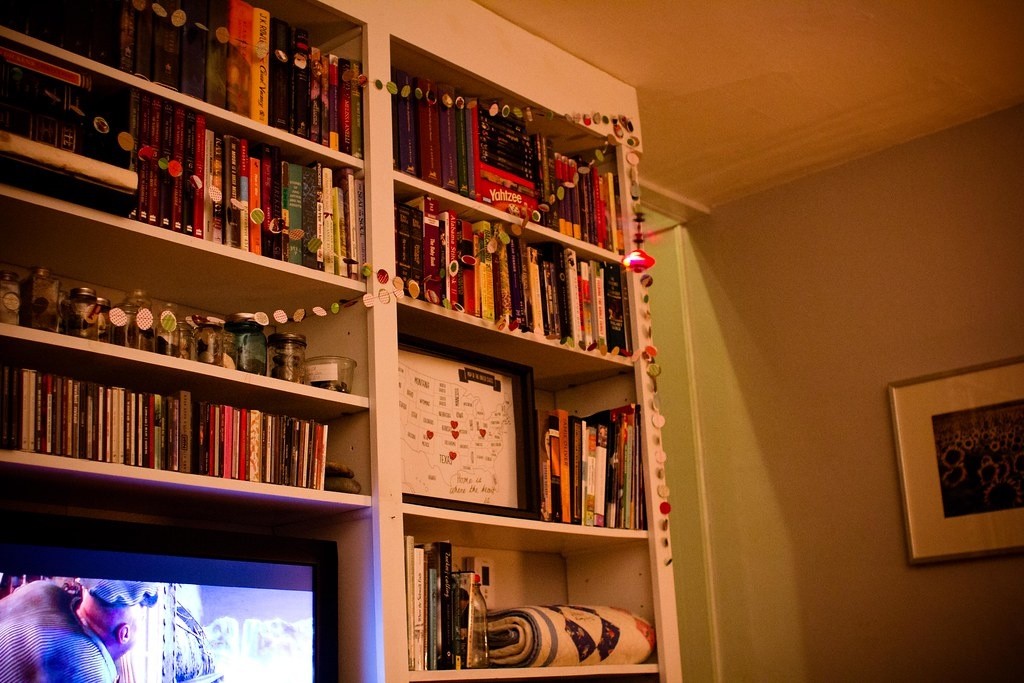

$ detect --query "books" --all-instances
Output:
[0,366,329,491]
[538,403,648,531]
[0,0,364,160]
[1,43,369,281]
[405,535,474,670]
[391,65,625,258]
[395,194,631,356]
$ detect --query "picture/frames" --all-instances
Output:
[888,356,1024,564]
[397,332,542,521]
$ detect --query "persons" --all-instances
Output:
[0,575,159,683]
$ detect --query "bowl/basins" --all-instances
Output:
[305,356,356,393]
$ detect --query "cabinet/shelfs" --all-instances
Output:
[0,0,684,683]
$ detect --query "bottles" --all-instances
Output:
[473,575,489,668]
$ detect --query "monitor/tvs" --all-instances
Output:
[0,510,339,683]
